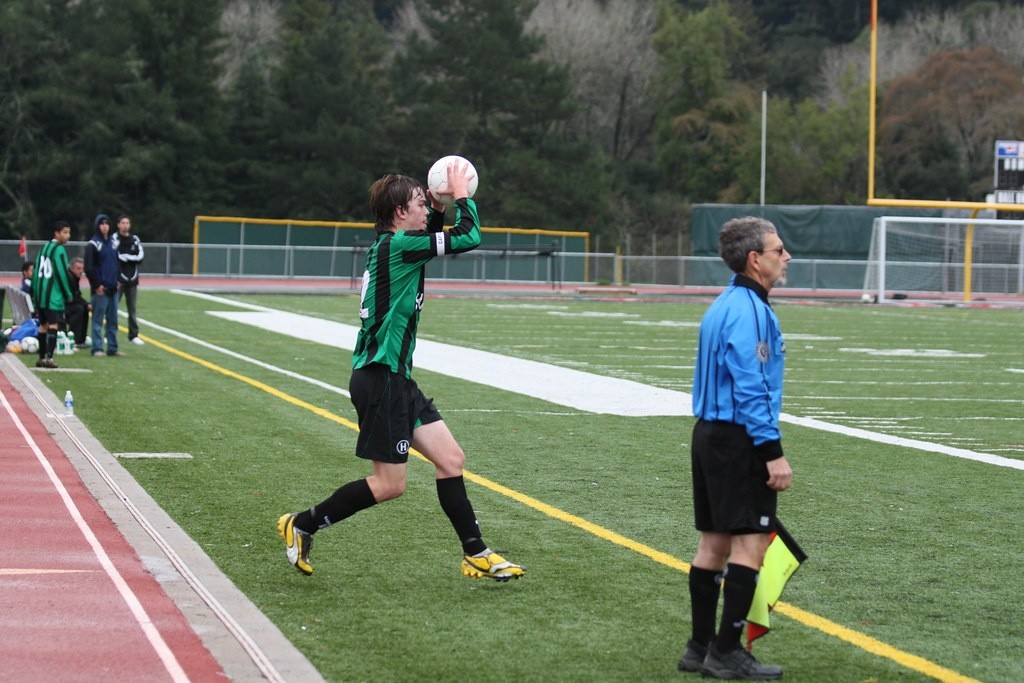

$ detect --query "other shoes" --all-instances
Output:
[131,336,144,346]
[75,343,89,349]
[115,351,125,356]
[94,351,104,356]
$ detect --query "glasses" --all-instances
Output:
[758,244,784,256]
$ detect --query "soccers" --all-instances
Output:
[429,155,479,200]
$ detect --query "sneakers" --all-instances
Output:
[700,637,783,679]
[461,549,527,582]
[35,357,58,368]
[677,638,708,671]
[278,512,313,575]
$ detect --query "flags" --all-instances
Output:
[746,531,799,651]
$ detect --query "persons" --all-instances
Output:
[112,216,144,345]
[277,159,526,583]
[22,262,34,293]
[85,214,126,356]
[677,216,793,678]
[31,222,74,368]
[65,257,91,348]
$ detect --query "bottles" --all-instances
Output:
[64,391,73,416]
[57,330,75,355]
